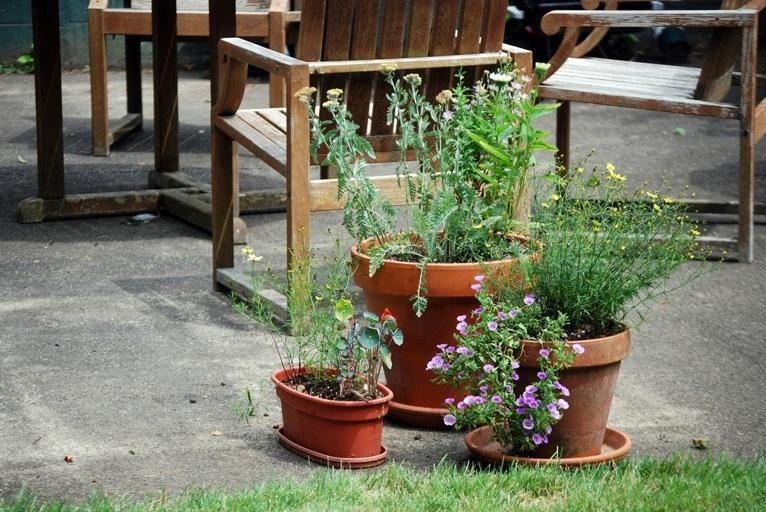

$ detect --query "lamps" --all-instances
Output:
[85,0,298,158]
[206,0,536,336]
[529,0,766,264]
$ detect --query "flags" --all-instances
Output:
[269,367,393,470]
[350,227,543,430]
[464,318,632,474]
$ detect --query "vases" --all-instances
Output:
[220,224,406,401]
[424,145,729,453]
[294,47,563,319]
